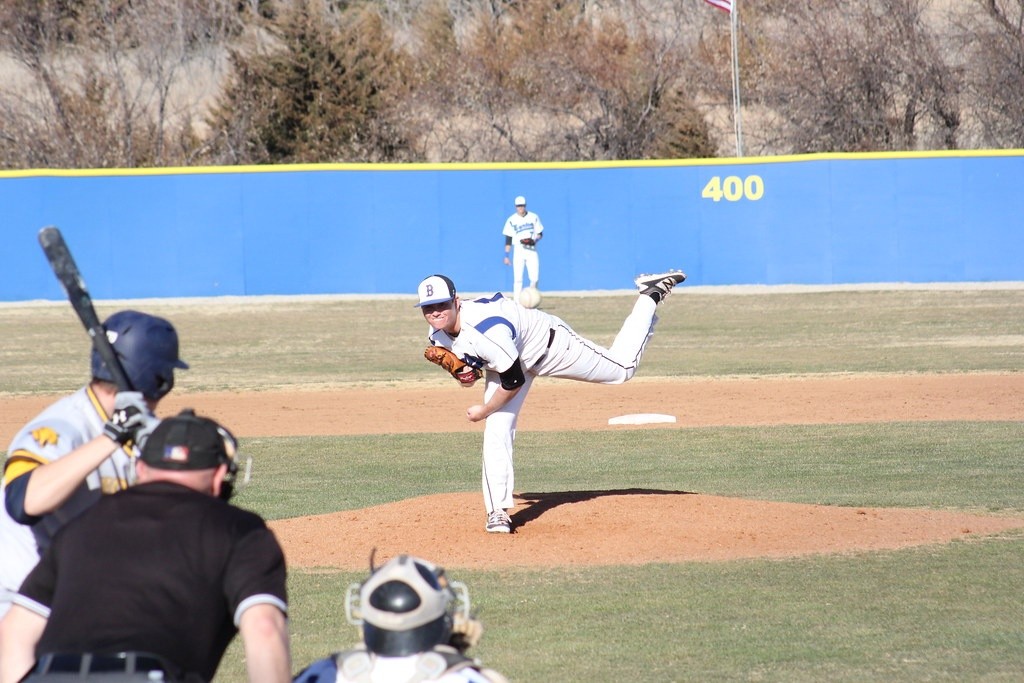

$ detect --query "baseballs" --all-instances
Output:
[520,286,542,309]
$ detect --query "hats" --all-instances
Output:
[141,416,226,471]
[414,274,456,308]
[515,196,526,206]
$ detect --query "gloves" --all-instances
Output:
[102,392,161,455]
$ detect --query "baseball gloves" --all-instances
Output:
[520,238,535,246]
[423,345,483,384]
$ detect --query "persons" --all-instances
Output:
[0,310,190,622]
[414,270,688,535]
[291,548,512,683]
[0,415,295,683]
[502,196,544,307]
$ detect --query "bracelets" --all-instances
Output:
[534,238,538,241]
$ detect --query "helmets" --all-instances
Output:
[363,561,443,658]
[91,311,189,401]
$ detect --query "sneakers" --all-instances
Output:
[486,509,513,534]
[634,269,686,305]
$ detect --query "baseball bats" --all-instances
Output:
[37,224,133,392]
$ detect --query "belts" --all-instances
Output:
[535,327,555,364]
[34,655,162,672]
